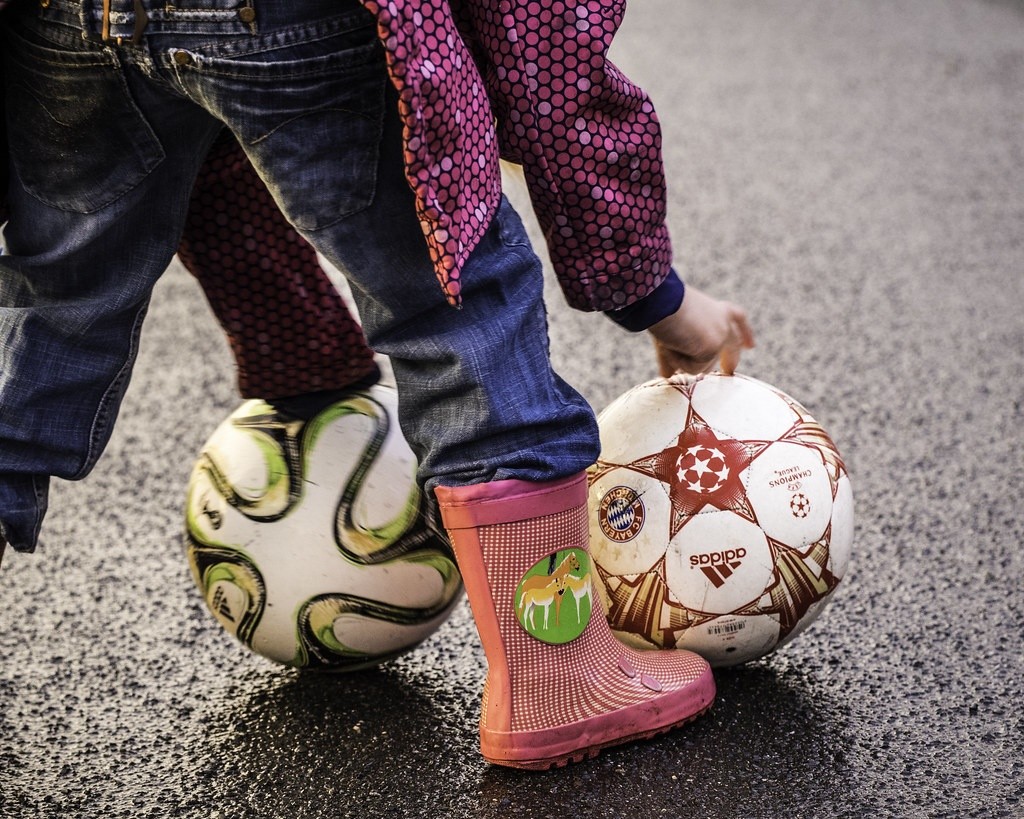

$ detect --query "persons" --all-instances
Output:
[1,0,754,772]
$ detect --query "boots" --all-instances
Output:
[435,468,718,772]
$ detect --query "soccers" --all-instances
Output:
[185,381,467,675]
[580,370,856,668]
